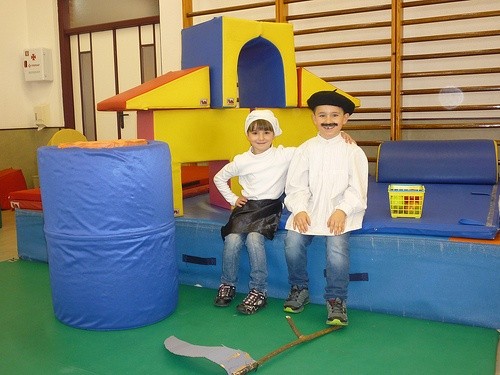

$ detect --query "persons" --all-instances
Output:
[284,90,368,324]
[212,109,358,316]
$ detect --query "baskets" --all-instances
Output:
[387,185,425,219]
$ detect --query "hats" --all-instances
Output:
[308,89,355,116]
[245,109,282,138]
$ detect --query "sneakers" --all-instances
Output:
[326,298,348,326]
[213,284,236,308]
[283,285,309,314]
[236,288,266,314]
